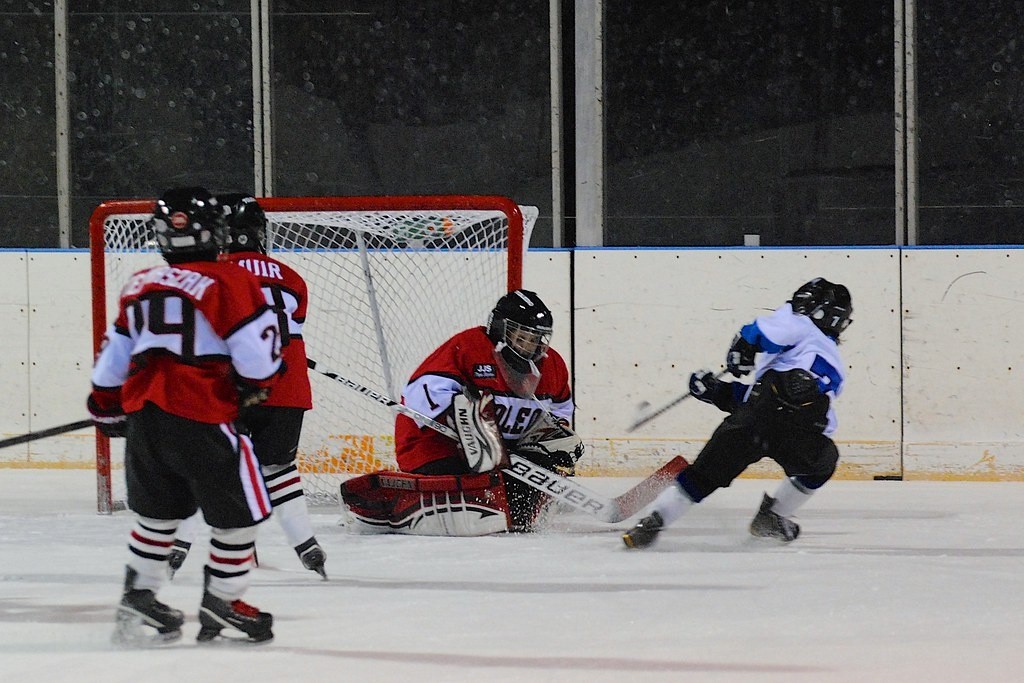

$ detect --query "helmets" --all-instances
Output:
[215,192,266,252]
[151,186,224,264]
[487,289,553,373]
[792,277,854,338]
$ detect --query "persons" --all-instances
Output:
[168,193,329,583]
[396,291,584,532]
[621,277,853,549]
[88,188,282,644]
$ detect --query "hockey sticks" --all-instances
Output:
[0,417,97,452]
[306,357,691,526]
[624,362,733,435]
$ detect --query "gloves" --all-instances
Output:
[689,370,725,404]
[727,331,756,378]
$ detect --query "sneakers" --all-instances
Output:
[196,591,274,646]
[168,539,192,581]
[623,511,664,550]
[112,588,186,646]
[750,509,800,544]
[294,537,328,579]
